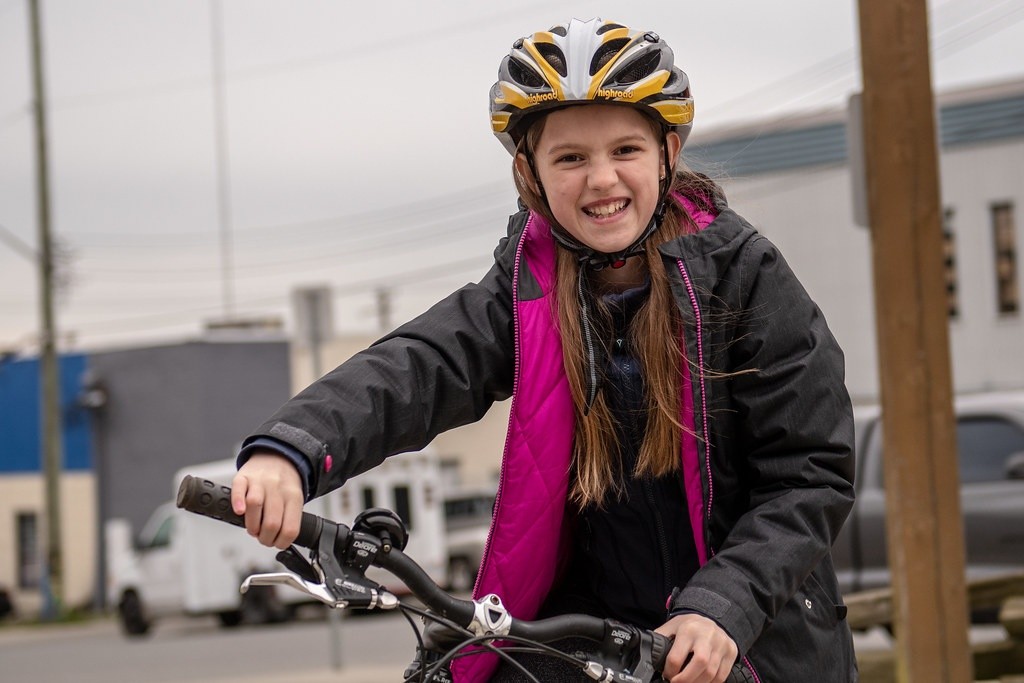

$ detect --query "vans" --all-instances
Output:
[118,449,445,637]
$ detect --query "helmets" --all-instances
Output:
[488,17,695,152]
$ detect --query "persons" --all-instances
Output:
[233,16,859,683]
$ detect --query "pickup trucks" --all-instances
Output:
[832,394,1024,650]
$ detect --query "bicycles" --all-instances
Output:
[176,475,754,683]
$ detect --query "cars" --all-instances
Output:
[440,478,499,593]
